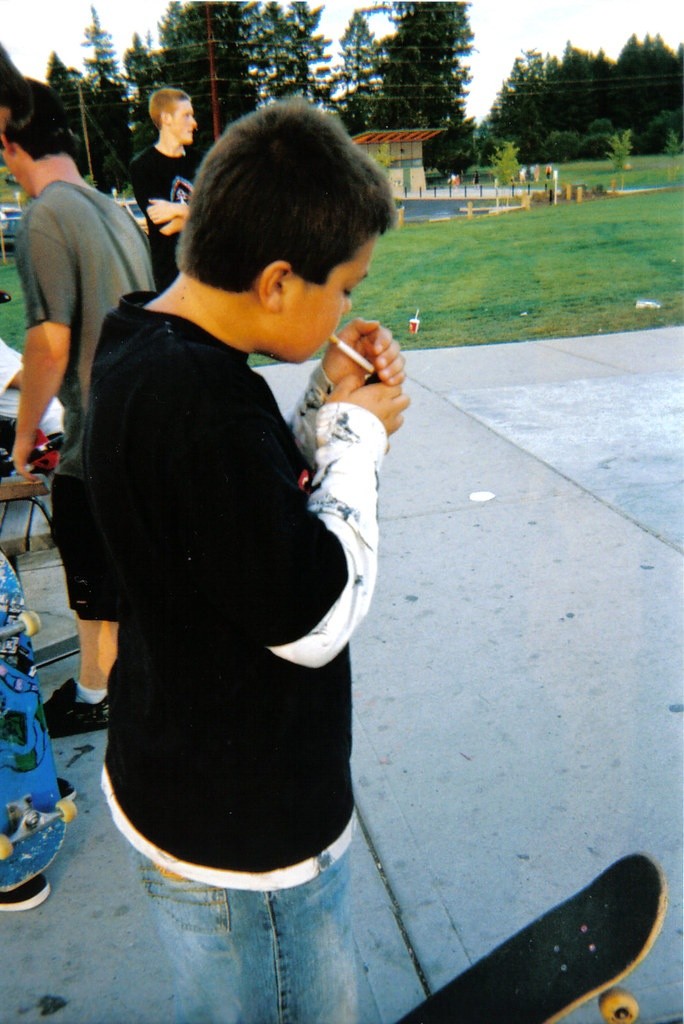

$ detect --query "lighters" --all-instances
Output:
[363,369,380,384]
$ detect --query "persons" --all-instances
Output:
[0,43,155,913]
[81,97,409,1024]
[132,85,213,292]
[451,164,553,189]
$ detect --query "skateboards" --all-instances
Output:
[388,849,669,1024]
[0,549,79,894]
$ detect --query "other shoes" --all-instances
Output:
[0,870,52,913]
[57,776,76,801]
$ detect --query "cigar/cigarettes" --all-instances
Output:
[331,333,376,375]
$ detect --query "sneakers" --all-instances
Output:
[41,678,111,739]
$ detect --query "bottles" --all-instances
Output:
[635,300,660,309]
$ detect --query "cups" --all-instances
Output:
[409,319,420,334]
